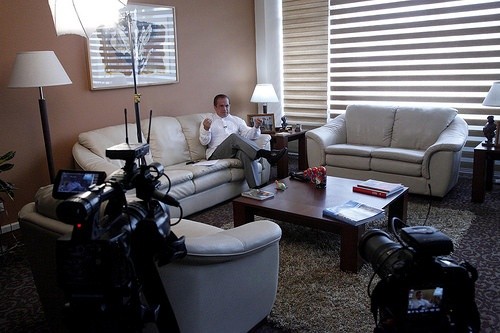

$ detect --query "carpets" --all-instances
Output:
[187,182,478,332]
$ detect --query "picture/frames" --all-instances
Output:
[247,113,275,134]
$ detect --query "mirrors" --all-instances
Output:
[84,2,180,91]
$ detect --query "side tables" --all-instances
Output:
[471,138,500,202]
[265,129,307,180]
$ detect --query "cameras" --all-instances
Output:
[358,228,472,333]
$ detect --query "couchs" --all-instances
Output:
[18,185,283,333]
[305,101,469,198]
[71,112,271,218]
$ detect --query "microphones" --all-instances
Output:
[152,191,181,207]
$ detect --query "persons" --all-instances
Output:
[199,94,288,189]
[66,176,86,192]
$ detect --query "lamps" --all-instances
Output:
[482,82,500,146]
[48,0,128,40]
[7,49,73,182]
[250,83,279,113]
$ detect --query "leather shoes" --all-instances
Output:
[268,147,288,166]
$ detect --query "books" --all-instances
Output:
[323,199,385,226]
[352,179,404,197]
[241,189,275,200]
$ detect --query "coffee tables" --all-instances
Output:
[232,169,411,273]
